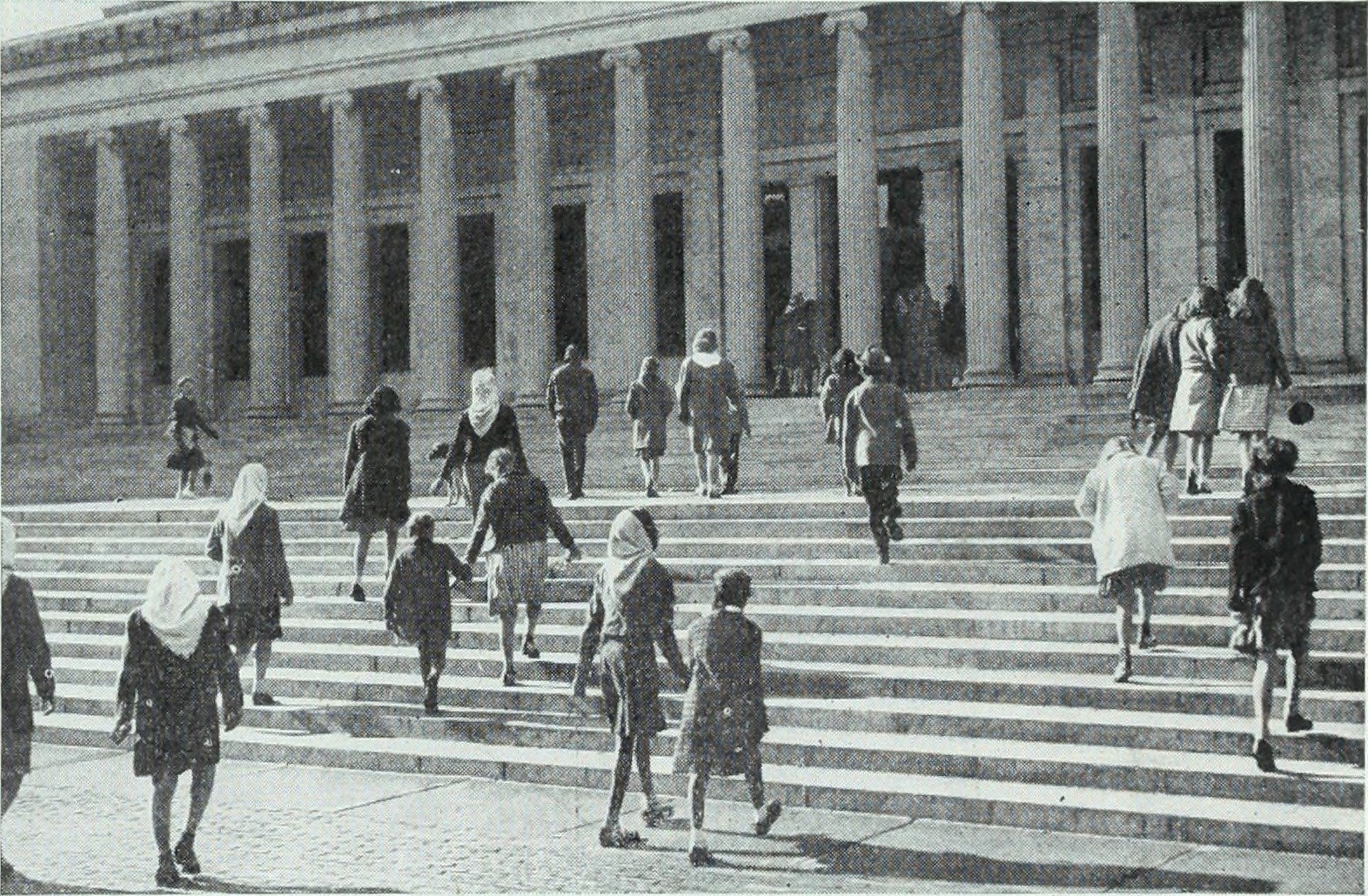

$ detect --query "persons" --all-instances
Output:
[677,329,751,497]
[0,516,54,820]
[546,344,599,500]
[109,559,243,886]
[166,377,222,500]
[572,508,691,845]
[430,367,528,531]
[625,357,674,497]
[383,513,471,712]
[819,347,874,496]
[674,568,780,864]
[843,346,918,563]
[463,448,579,685]
[1230,437,1323,772]
[339,385,412,602]
[1129,276,1294,495]
[1074,435,1176,681]
[206,463,293,705]
[770,282,972,396]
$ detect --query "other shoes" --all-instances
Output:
[154,866,183,886]
[1137,632,1163,649]
[751,796,786,835]
[349,584,367,602]
[182,486,201,500]
[1284,712,1313,732]
[1183,475,1199,495]
[1196,483,1213,494]
[423,679,439,712]
[521,637,541,659]
[174,843,204,873]
[1251,738,1279,772]
[251,691,280,706]
[176,491,187,500]
[1112,664,1137,682]
[599,824,648,850]
[687,844,716,869]
[644,802,677,827]
[500,669,521,688]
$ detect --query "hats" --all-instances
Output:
[854,345,895,372]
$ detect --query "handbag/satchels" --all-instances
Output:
[1283,397,1317,427]
[165,445,194,473]
